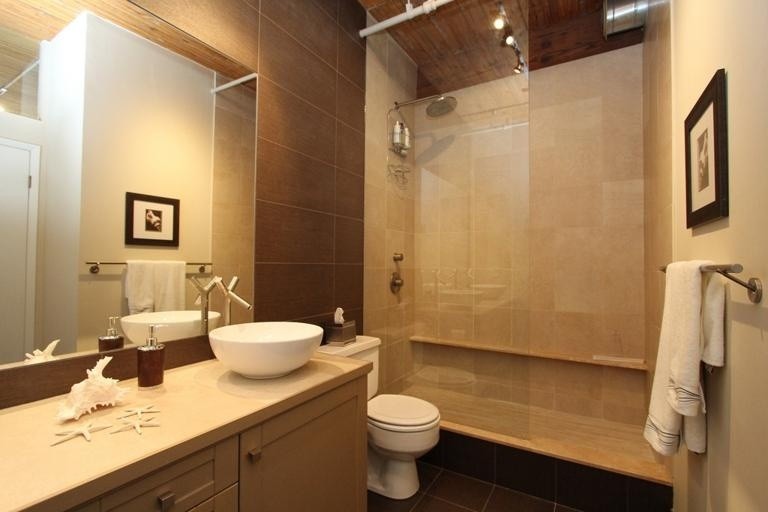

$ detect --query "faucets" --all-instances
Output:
[189,276,216,335]
[216,275,252,327]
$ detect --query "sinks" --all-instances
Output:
[208,320,324,379]
[120,309,221,341]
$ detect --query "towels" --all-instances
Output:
[642,261,707,460]
[154,260,185,314]
[660,259,726,419]
[123,260,154,315]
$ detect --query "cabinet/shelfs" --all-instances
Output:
[239,378,367,512]
[100,436,239,511]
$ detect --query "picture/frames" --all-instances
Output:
[684,68,729,229]
[125,192,180,247]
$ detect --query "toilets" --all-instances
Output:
[316,336,440,500]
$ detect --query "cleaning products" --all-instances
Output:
[136,324,166,391]
[98,316,125,352]
